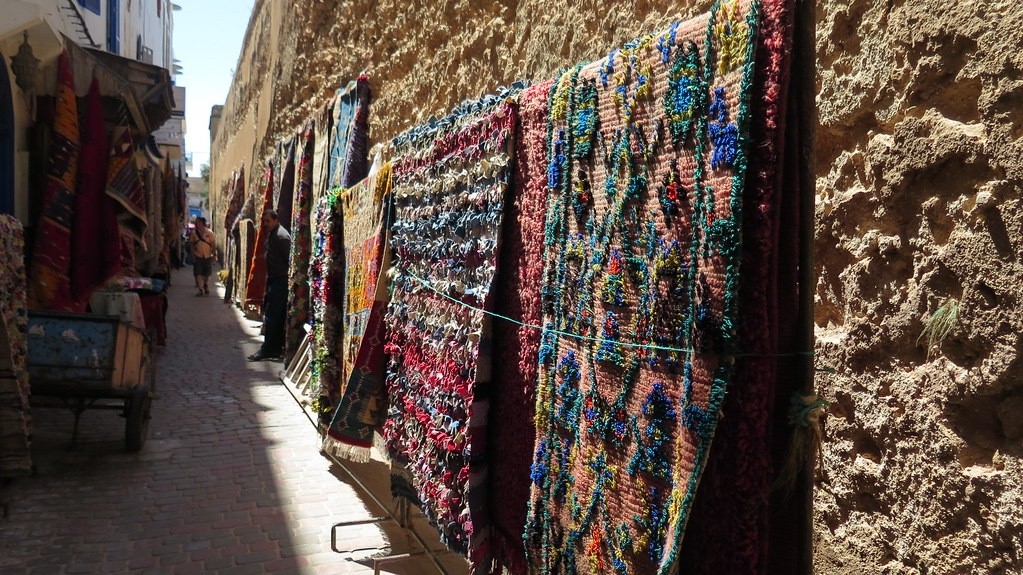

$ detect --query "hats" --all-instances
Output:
[197,217,207,228]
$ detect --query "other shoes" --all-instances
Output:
[203,284,210,294]
[247,350,280,360]
[195,289,204,297]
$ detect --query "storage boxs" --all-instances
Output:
[27,311,149,390]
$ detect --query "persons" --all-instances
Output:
[189,217,215,297]
[181,237,187,268]
[246,209,291,362]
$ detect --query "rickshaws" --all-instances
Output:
[22,307,159,452]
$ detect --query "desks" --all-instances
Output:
[91,278,167,347]
